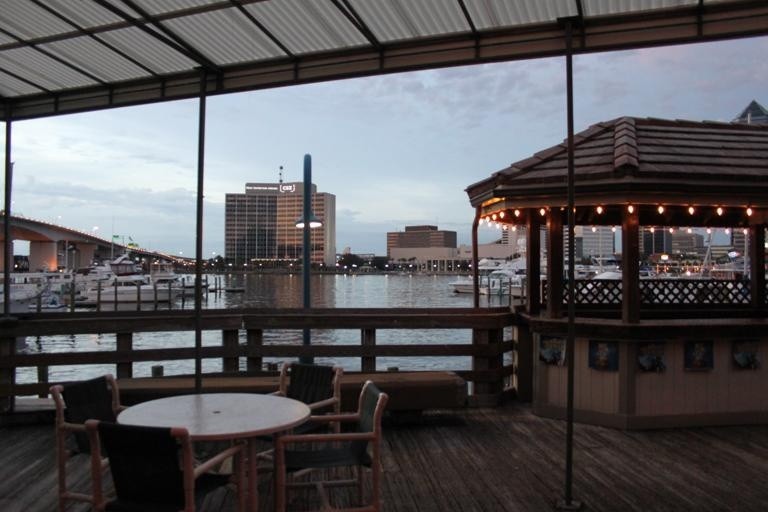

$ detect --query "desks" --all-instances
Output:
[116,393,311,512]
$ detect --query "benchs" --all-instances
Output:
[113,370,468,417]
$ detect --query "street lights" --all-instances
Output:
[293,154,325,362]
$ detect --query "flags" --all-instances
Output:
[112,234,119,239]
[128,242,138,248]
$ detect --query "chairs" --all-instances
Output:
[272,379,387,511]
[256,363,344,464]
[51,373,131,503]
[84,418,247,512]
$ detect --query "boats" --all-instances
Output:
[447,254,707,303]
[0,262,209,305]
